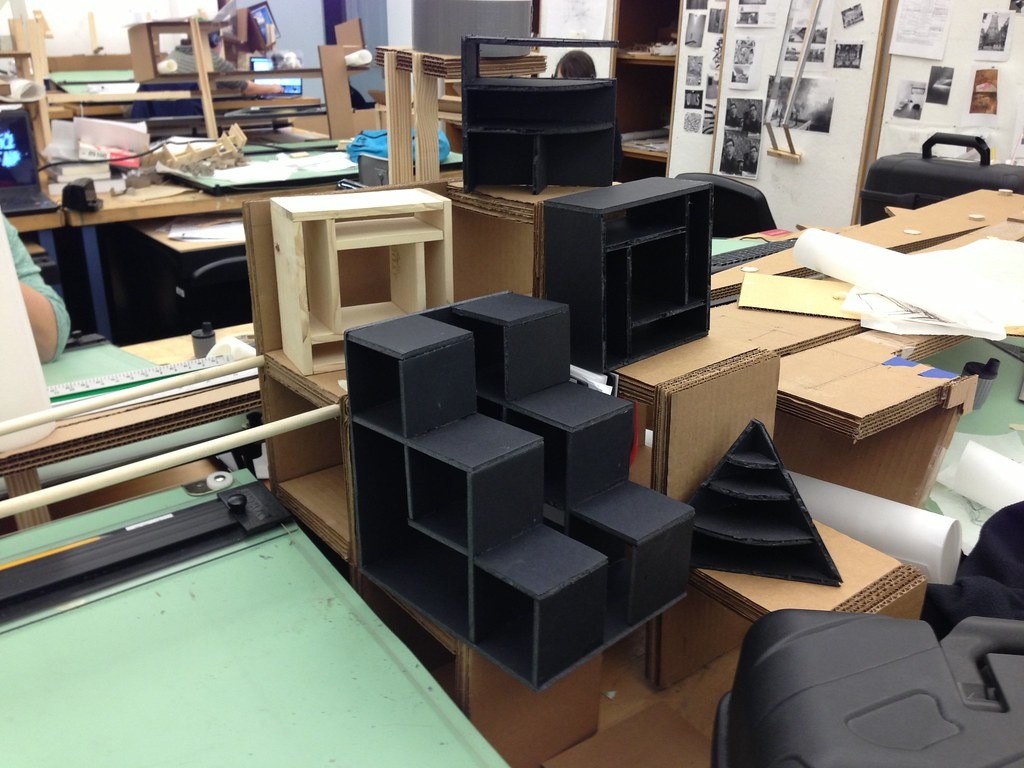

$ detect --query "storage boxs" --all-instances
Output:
[712,610,1024,768]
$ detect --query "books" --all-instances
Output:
[36,134,142,200]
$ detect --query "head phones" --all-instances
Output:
[209,31,220,48]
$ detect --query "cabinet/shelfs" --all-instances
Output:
[608,0,680,180]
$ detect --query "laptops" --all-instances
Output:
[0,110,59,216]
[249,57,302,98]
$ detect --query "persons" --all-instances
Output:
[551,50,624,186]
[2,206,71,364]
[721,101,761,174]
[162,20,286,115]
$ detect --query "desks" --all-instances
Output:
[0,324,272,533]
[921,335,1024,551]
[56,93,321,117]
[43,126,464,346]
[6,210,68,303]
